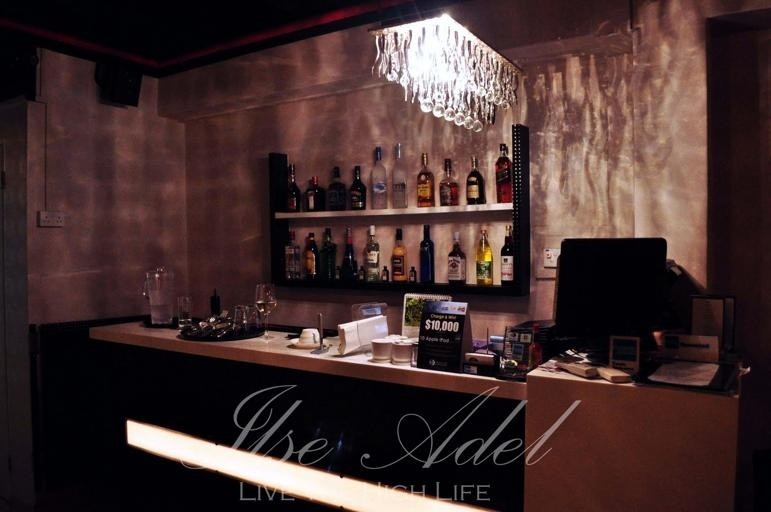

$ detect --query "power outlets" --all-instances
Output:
[37,210,64,227]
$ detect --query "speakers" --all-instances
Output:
[94,56,144,107]
[1,47,39,101]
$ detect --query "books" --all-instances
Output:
[401,293,453,339]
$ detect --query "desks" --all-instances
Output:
[524,354,742,512]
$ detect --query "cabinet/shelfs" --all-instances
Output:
[268,122,531,297]
[706,6,771,512]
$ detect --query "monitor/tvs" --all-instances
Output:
[554,237,668,342]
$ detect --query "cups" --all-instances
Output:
[370,333,423,364]
[299,328,321,344]
[143,267,175,325]
[177,295,194,328]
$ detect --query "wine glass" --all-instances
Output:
[255,282,278,342]
[235,302,257,331]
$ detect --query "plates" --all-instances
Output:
[291,337,328,348]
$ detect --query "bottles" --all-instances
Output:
[500,352,517,377]
[286,142,513,211]
[282,224,517,284]
[527,323,543,368]
[209,287,221,315]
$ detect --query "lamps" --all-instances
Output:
[367,7,524,133]
